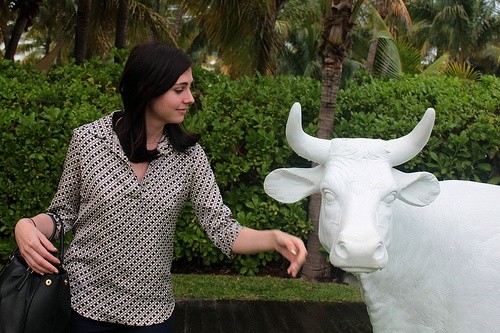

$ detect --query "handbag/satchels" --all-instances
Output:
[0,211,70,332]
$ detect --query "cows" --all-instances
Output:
[263,101,499,332]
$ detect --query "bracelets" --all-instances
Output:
[28,217,37,228]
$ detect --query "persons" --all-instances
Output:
[12,40,308,332]
